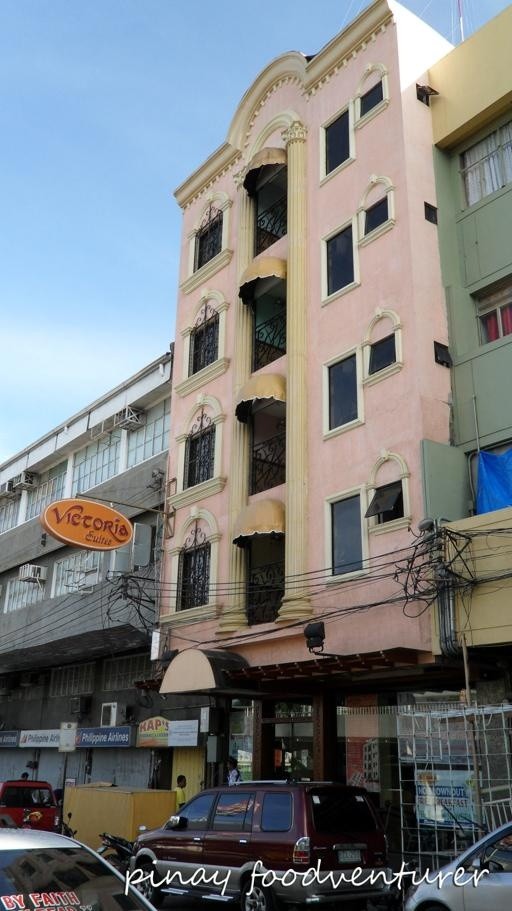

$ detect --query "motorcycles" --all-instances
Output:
[96,825,151,875]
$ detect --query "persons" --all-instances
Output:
[172,775,187,814]
[225,758,242,788]
[20,772,30,782]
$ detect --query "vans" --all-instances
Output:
[0,780,62,833]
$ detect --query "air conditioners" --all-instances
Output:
[119,411,146,431]
[0,472,39,501]
[100,702,126,728]
[20,566,47,582]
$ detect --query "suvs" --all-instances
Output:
[129,779,392,911]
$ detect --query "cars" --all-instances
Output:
[405,820,512,910]
[1,827,158,910]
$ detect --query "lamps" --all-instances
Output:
[303,622,344,657]
[160,649,178,669]
